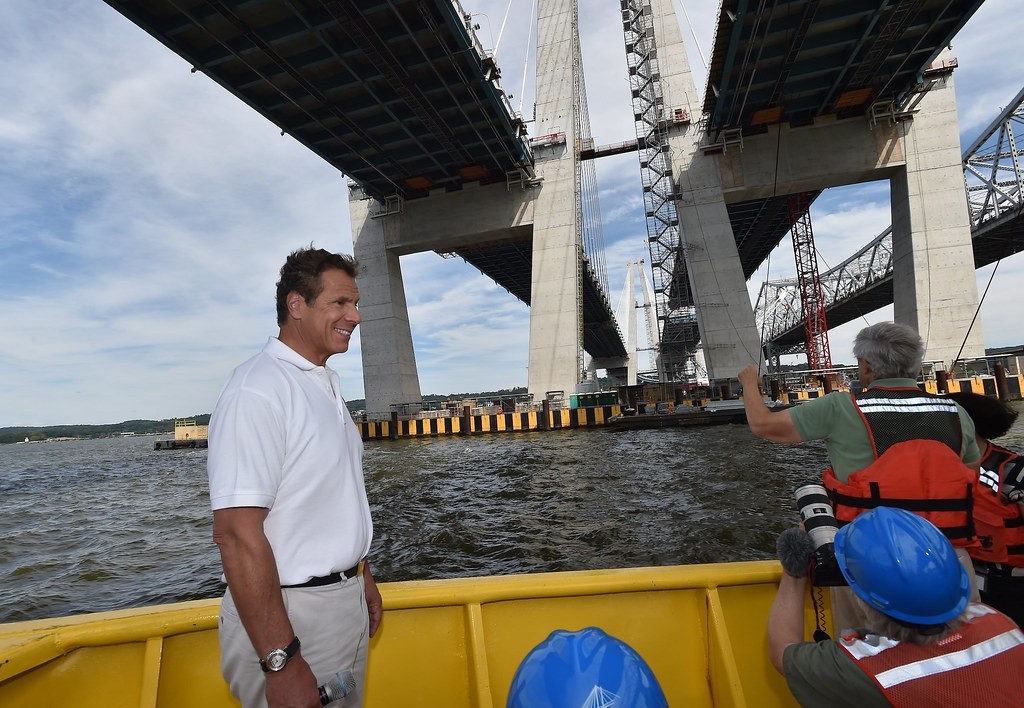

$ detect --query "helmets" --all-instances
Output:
[506,626,668,708]
[834,506,971,625]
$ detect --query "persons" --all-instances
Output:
[737,322,981,641]
[943,391,1024,634]
[206,246,383,708]
[766,505,1024,708]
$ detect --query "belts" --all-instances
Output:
[281,564,358,588]
[972,558,1024,577]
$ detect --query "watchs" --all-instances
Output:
[259,635,301,673]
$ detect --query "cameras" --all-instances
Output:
[793,484,850,588]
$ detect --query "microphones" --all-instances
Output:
[776,527,817,578]
